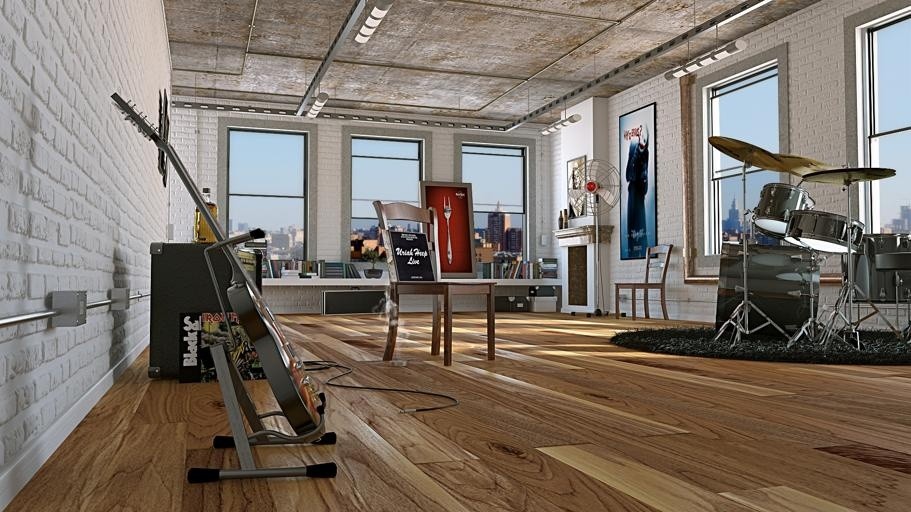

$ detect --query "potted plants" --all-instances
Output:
[362,246,385,279]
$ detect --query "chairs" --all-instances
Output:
[370,197,499,363]
[612,244,671,321]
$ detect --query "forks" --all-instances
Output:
[442,196,454,265]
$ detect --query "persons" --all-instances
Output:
[569,167,585,218]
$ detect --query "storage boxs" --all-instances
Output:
[529,287,555,298]
[529,296,559,313]
[497,295,527,312]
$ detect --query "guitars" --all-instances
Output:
[111,92,321,441]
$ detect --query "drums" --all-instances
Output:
[753,183,810,242]
[839,233,910,305]
[785,209,863,254]
[714,242,821,333]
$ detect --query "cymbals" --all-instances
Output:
[803,165,897,185]
[707,134,785,175]
[777,150,829,176]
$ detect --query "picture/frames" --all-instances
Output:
[619,103,657,259]
[420,181,477,276]
[566,155,587,219]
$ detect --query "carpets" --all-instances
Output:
[607,322,911,370]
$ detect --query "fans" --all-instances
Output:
[567,159,627,318]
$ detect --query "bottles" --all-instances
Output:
[563,209,569,228]
[193,187,218,243]
[558,210,563,230]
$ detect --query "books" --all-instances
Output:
[482,257,558,278]
[245,240,268,278]
[269,259,319,279]
[320,259,361,278]
[237,249,256,272]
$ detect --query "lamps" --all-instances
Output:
[664,39,752,78]
[297,90,329,125]
[352,4,388,46]
[539,111,579,137]
